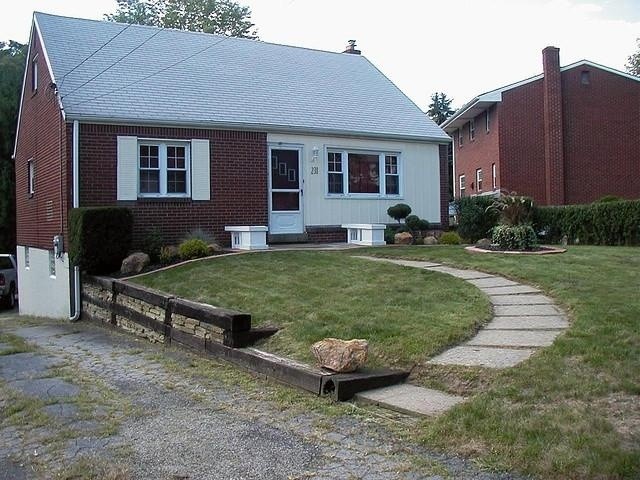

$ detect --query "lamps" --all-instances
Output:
[309,145,319,163]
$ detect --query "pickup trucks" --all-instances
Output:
[0,254,18,309]
